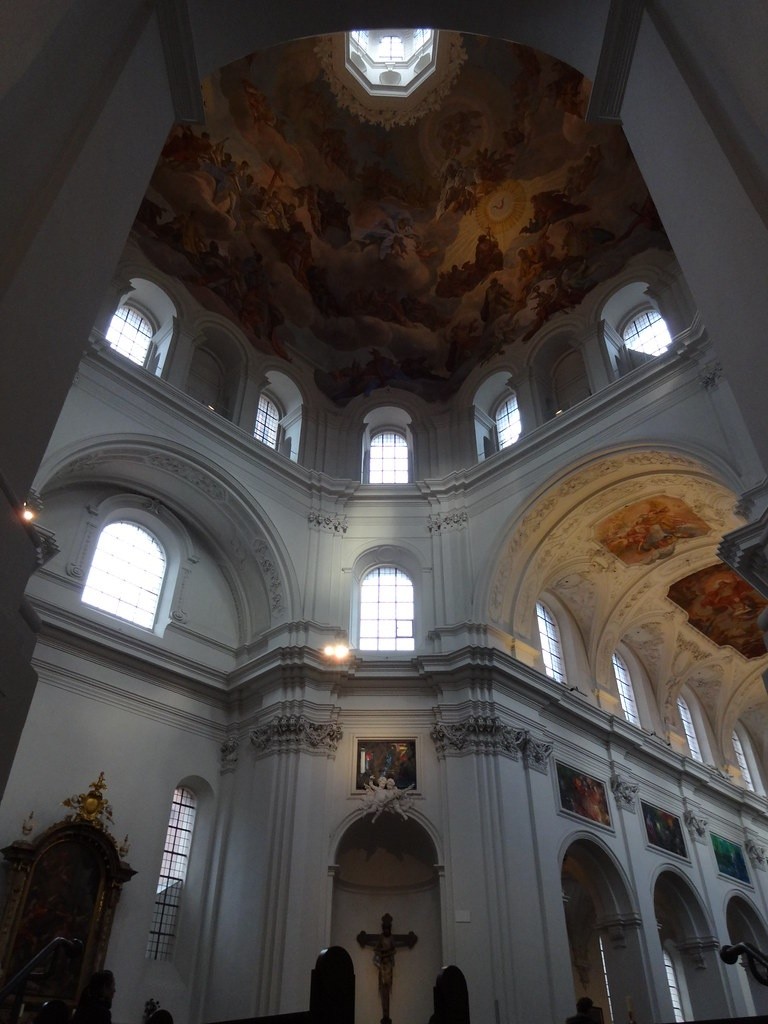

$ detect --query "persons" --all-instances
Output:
[363,776,414,823]
[146,1010,173,1024]
[373,913,396,1024]
[34,970,116,1024]
[566,997,598,1024]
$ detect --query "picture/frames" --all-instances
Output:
[550,754,616,837]
[707,827,756,893]
[638,795,692,867]
[347,733,424,799]
